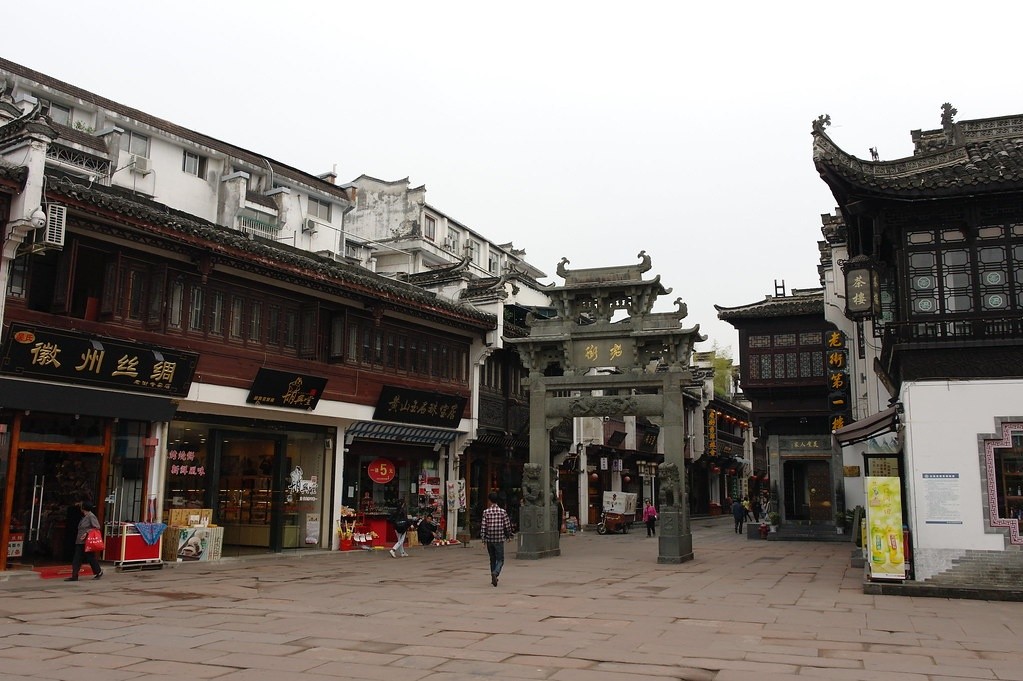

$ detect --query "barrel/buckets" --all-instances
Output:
[340,538,352,551]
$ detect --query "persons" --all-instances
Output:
[64,503,103,582]
[732,496,771,534]
[389,500,411,558]
[480,494,514,587]
[417,515,437,545]
[643,501,658,538]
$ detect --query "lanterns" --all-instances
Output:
[624,476,630,483]
[591,473,598,481]
[729,469,735,474]
[752,476,757,479]
[712,467,719,474]
[717,412,748,428]
[763,477,768,481]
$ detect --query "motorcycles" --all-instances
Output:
[597,506,637,535]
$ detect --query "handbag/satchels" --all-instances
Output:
[648,515,657,522]
[85,527,106,552]
[395,521,407,533]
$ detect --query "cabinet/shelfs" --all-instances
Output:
[219,472,304,549]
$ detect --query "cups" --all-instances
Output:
[183,531,208,556]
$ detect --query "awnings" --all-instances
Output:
[831,404,896,453]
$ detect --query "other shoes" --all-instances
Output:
[389,549,396,558]
[64,576,78,581]
[93,570,104,580]
[491,572,499,587]
[401,551,408,557]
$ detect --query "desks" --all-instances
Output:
[163,527,224,562]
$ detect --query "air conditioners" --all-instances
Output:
[131,154,153,173]
[466,248,474,258]
[445,238,452,246]
[304,218,319,232]
[503,261,510,269]
[466,237,474,248]
[26,201,68,249]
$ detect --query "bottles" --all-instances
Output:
[1008,487,1013,496]
[886,519,903,564]
[1010,506,1023,521]
[1018,486,1021,496]
[872,520,887,564]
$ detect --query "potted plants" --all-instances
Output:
[834,512,847,535]
[768,511,782,533]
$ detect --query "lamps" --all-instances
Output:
[344,433,355,446]
[433,440,442,452]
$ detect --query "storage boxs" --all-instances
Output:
[168,509,218,528]
[602,491,638,514]
